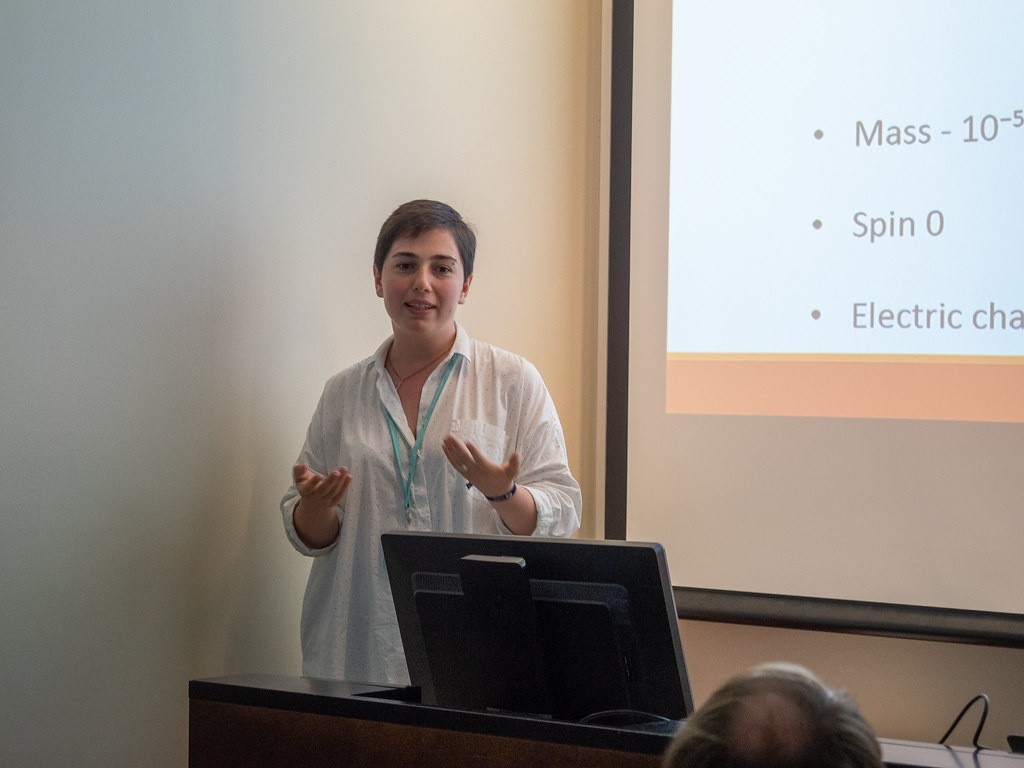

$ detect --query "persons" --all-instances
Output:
[280,197,584,691]
[661,660,884,768]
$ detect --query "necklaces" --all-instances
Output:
[388,351,448,392]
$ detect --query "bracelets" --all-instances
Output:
[484,482,517,501]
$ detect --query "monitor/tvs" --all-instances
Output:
[381,530,695,733]
